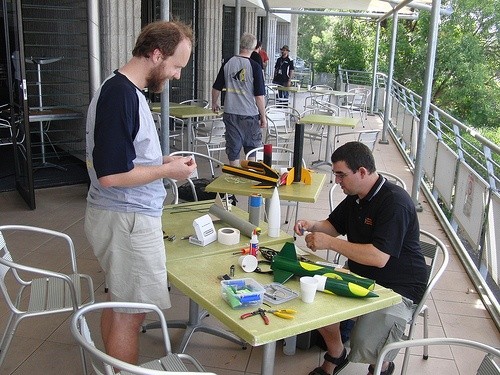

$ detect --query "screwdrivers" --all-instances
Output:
[233,248,250,255]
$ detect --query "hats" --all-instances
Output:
[280,45,290,52]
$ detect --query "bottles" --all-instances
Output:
[249,235,259,258]
[262,143,272,167]
[248,196,262,228]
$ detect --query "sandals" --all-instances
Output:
[308,347,349,375]
[367,361,395,375]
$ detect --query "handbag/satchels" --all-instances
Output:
[178,178,225,201]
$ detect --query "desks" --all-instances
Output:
[308,89,356,111]
[28,109,83,172]
[149,102,196,146]
[272,86,310,113]
[299,114,359,167]
[167,243,403,375]
[204,168,326,222]
[154,106,219,155]
[143,199,297,354]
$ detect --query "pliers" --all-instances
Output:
[266,309,297,319]
[241,307,270,325]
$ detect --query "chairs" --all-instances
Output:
[0,103,60,158]
[72,83,500,375]
[0,225,95,375]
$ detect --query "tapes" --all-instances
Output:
[218,228,240,245]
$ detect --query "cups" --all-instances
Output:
[238,255,258,272]
[300,276,317,303]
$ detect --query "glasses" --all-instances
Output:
[333,167,357,178]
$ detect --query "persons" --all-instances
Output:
[293,141,426,375]
[212,33,268,166]
[84,21,198,374]
[251,41,269,70]
[272,45,294,108]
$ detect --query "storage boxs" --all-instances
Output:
[221,278,265,309]
